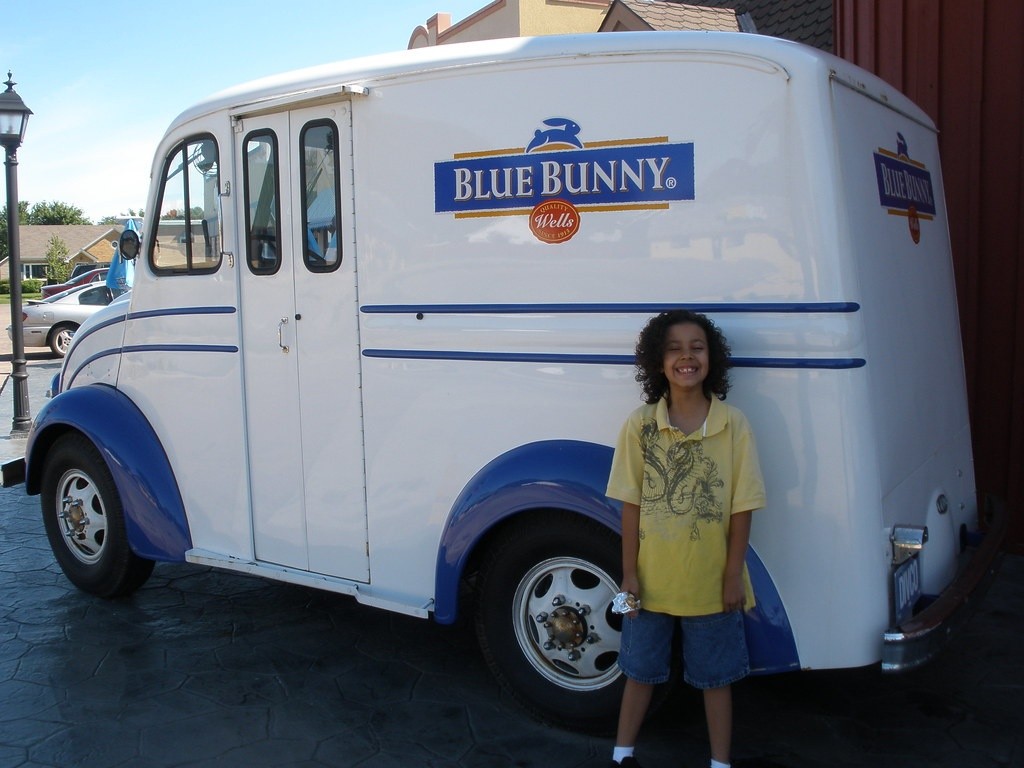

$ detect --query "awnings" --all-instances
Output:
[307,189,338,230]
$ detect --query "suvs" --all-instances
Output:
[71,261,112,279]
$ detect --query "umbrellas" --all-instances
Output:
[105,219,140,290]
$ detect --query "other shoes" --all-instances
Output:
[608,756,640,768]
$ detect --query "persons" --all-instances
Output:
[605,309,765,768]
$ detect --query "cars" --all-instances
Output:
[7,280,132,359]
[39,266,110,301]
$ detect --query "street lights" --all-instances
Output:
[0,71,39,437]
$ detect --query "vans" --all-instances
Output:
[21,32,988,736]
